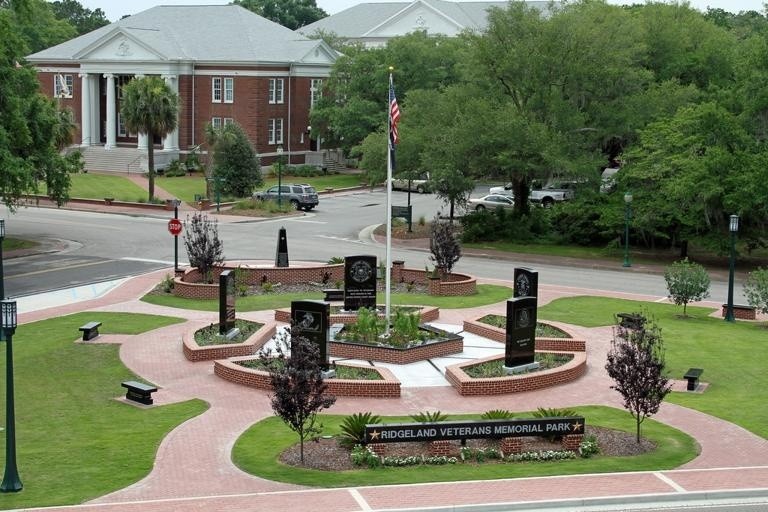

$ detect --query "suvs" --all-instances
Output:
[254,182,319,212]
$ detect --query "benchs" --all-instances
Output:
[616,310,645,330]
[320,286,344,302]
[77,319,103,341]
[683,365,706,391]
[121,380,159,405]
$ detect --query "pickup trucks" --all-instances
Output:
[490,181,569,207]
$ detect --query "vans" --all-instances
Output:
[383,170,434,192]
[605,168,628,194]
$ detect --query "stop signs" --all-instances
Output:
[167,219,181,234]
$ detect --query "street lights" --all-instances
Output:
[622,191,634,268]
[0,217,7,301]
[724,213,741,322]
[1,296,23,493]
[276,145,284,213]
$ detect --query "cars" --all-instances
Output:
[466,194,516,213]
[549,179,588,198]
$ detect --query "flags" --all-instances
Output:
[388,78,400,143]
[59,74,69,98]
